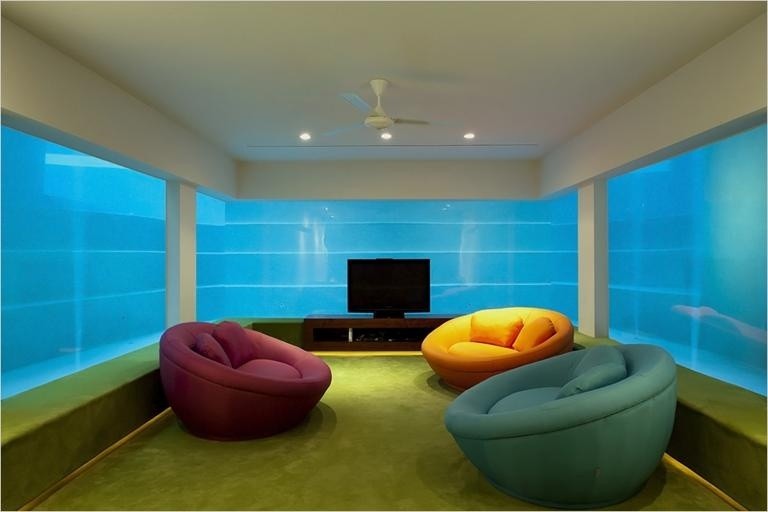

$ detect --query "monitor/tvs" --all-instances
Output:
[347,258,431,319]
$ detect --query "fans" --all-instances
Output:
[321,77,430,139]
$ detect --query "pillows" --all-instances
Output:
[511,316,555,351]
[471,310,523,348]
[212,321,258,368]
[561,346,626,387]
[196,333,232,368]
[555,362,628,399]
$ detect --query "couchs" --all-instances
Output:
[421,307,575,391]
[159,320,332,441]
[444,343,678,509]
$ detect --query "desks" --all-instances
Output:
[304,314,463,352]
